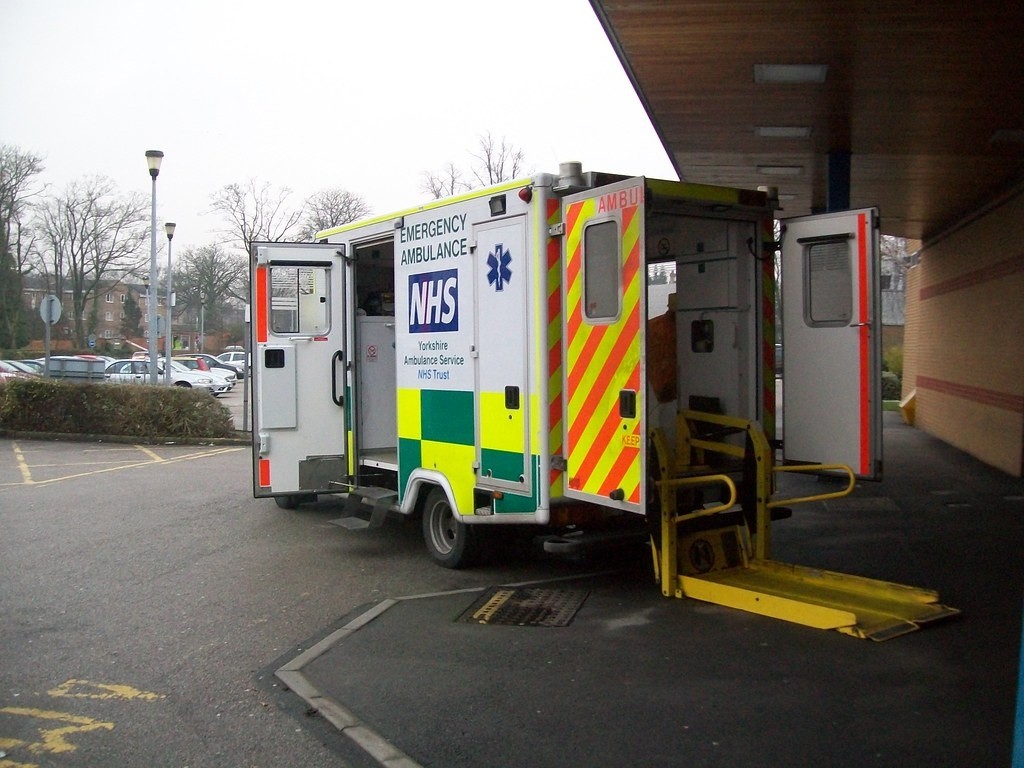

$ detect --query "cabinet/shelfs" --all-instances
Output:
[356,316,398,455]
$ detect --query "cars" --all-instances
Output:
[0,345,251,398]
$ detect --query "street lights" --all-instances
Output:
[145,150,164,384]
[165,223,176,386]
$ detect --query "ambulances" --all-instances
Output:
[249,161,886,570]
